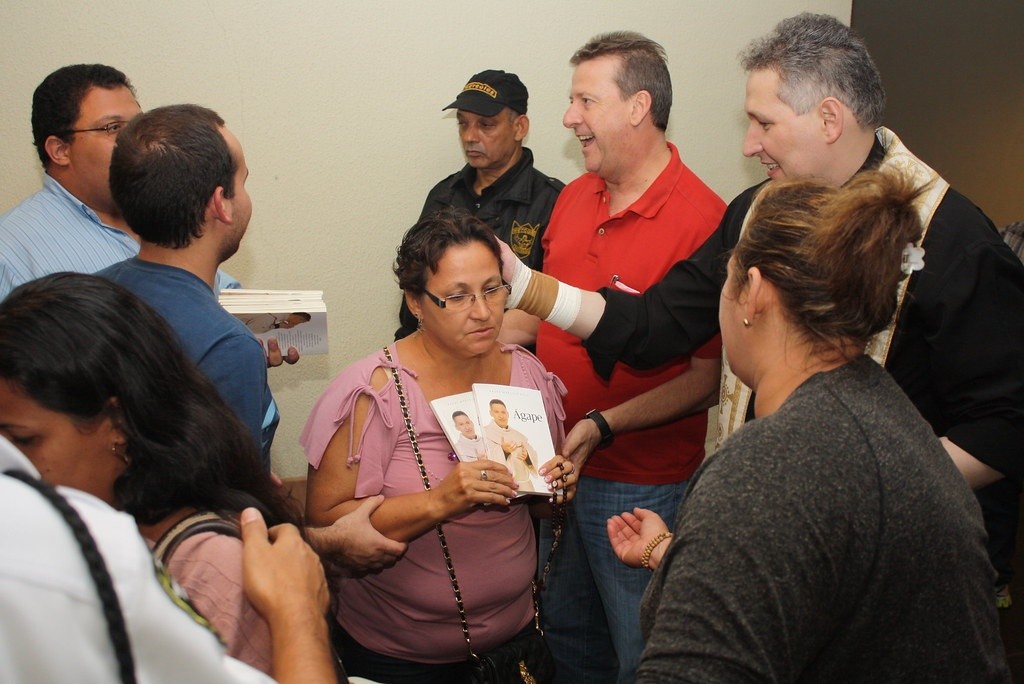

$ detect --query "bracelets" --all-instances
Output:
[584,409,614,449]
[640,532,674,569]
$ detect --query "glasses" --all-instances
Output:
[422,279,512,312]
[74,120,130,141]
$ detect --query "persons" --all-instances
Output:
[90,103,286,487]
[450,410,488,479]
[0,63,300,367]
[0,434,340,684]
[301,209,577,684]
[394,70,567,342]
[497,30,730,683]
[233,312,312,333]
[0,272,307,681]
[605,170,1019,684]
[491,11,1024,684]
[484,397,539,492]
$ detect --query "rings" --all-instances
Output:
[480,469,487,480]
[571,465,575,473]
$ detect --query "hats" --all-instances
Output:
[442,70,528,117]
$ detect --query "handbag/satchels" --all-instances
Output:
[469,632,555,684]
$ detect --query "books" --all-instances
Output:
[216,287,330,356]
[429,383,564,506]
